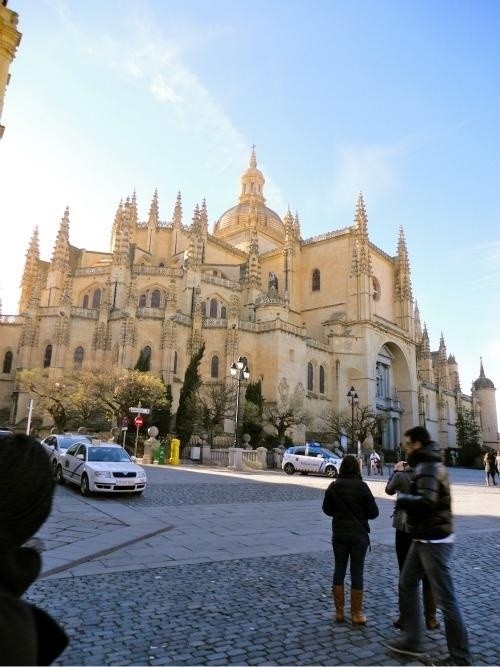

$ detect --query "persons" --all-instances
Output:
[494,451,500,477]
[483,453,496,487]
[391,426,476,665]
[369,450,380,476]
[320,456,379,625]
[384,460,441,630]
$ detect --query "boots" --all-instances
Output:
[351,589,367,625]
[331,584,344,623]
[423,590,440,630]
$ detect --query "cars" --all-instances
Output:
[0,426,15,434]
[39,432,94,473]
[281,441,343,477]
[56,441,147,496]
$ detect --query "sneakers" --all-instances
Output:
[432,657,459,666]
[383,637,426,657]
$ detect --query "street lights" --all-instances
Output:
[230,354,250,447]
[347,385,359,452]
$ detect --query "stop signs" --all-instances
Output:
[123,417,129,426]
[134,415,143,426]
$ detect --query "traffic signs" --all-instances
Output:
[129,406,150,414]
[121,426,128,430]
